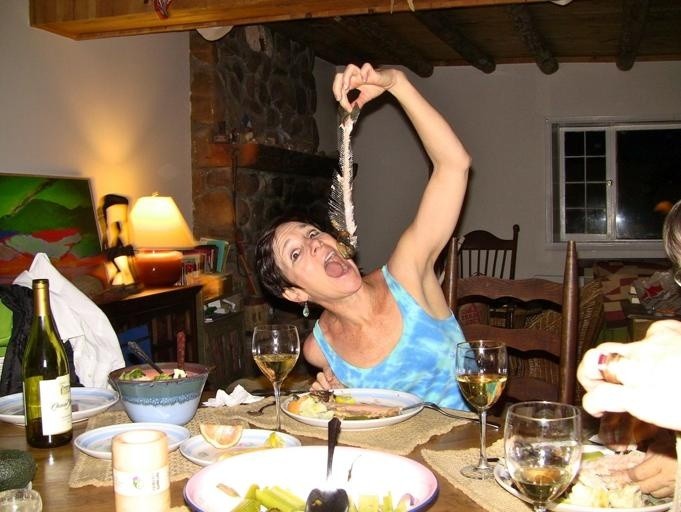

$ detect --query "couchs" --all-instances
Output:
[575,257,679,344]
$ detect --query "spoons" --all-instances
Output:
[305,416,350,511]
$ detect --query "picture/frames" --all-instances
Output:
[0,171,106,285]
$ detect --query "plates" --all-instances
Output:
[180,428,302,467]
[278,388,425,430]
[493,443,677,512]
[73,422,190,461]
[0,386,120,428]
[179,445,438,511]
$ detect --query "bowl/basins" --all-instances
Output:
[109,362,214,424]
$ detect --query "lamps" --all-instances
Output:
[123,191,199,287]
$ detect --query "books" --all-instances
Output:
[183,238,232,276]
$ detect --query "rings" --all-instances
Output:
[599,350,626,385]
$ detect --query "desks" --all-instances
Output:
[84,280,206,366]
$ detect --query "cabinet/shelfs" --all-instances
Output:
[192,271,249,390]
[619,298,681,345]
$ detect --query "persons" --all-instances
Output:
[576,317,681,440]
[595,198,681,502]
[254,59,494,415]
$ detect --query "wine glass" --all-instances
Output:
[503,400,583,512]
[250,323,302,434]
[455,339,510,481]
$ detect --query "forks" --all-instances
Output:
[397,401,499,435]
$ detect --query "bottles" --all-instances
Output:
[21,278,75,449]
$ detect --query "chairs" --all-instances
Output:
[444,232,582,432]
[439,223,524,332]
